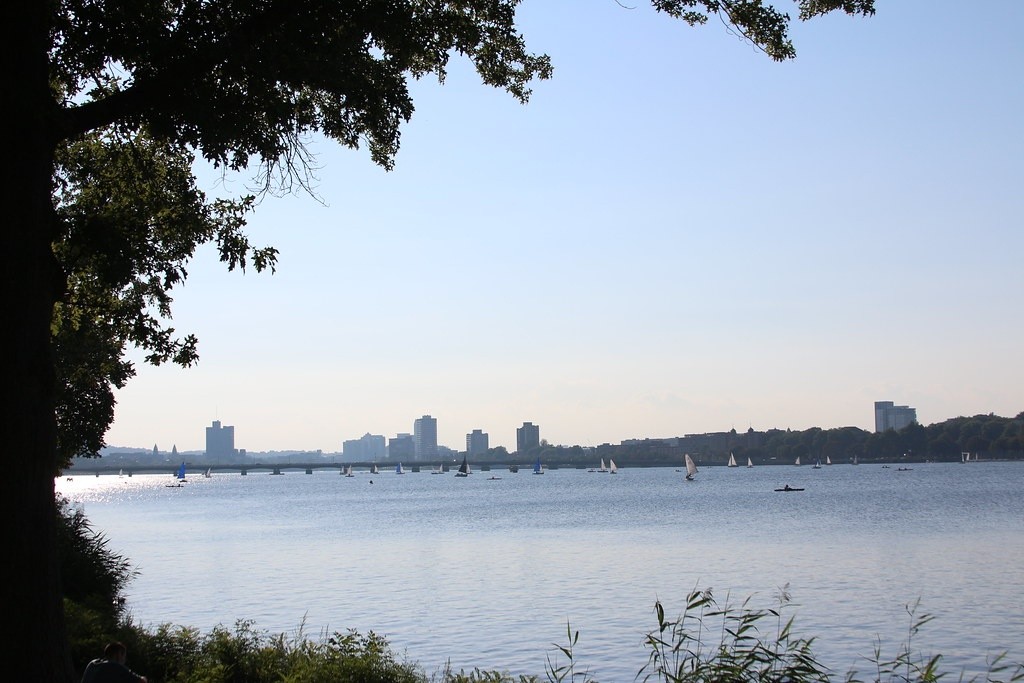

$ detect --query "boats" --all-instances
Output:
[774,488,805,492]
[813,461,822,469]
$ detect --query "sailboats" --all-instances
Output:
[173,461,187,482]
[684,454,698,480]
[340,465,355,477]
[728,452,738,467]
[430,464,444,474]
[118,468,124,478]
[396,463,406,474]
[206,465,214,479]
[747,457,754,469]
[369,465,380,474]
[587,458,617,474]
[826,456,831,465]
[795,457,801,466]
[456,456,473,477]
[533,456,544,474]
